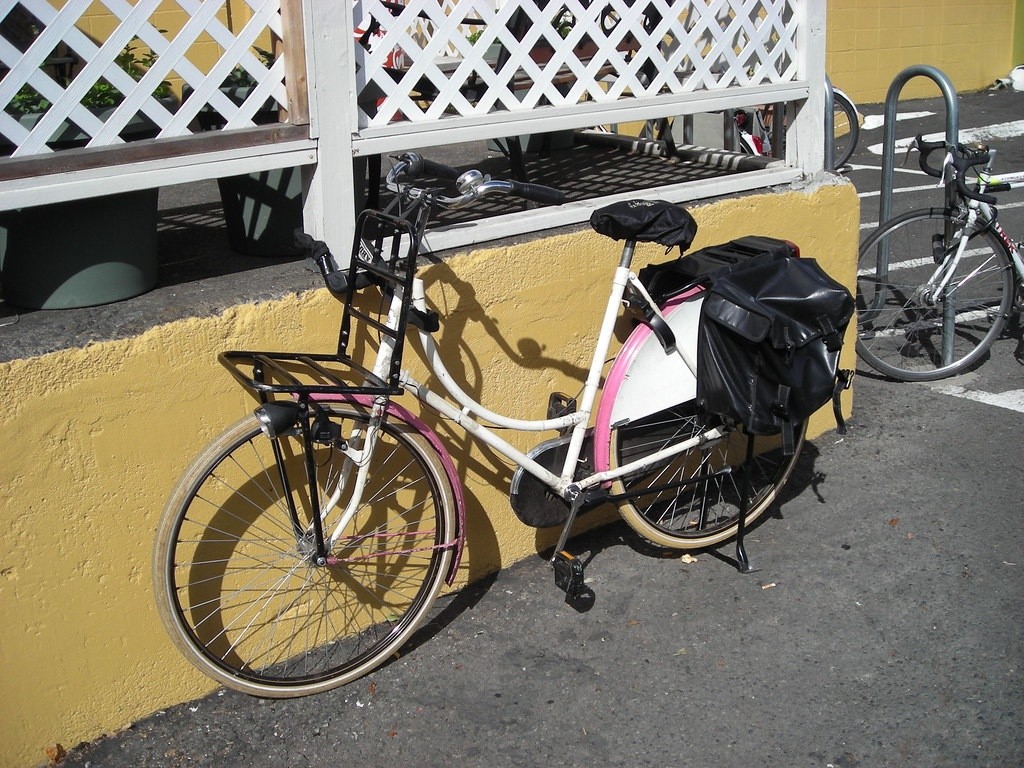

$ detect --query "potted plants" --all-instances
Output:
[0,35,177,144]
[182,46,278,112]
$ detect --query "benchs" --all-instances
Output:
[353,0,682,187]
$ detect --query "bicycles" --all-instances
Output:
[609,56,860,170]
[149,148,845,699]
[854,134,1023,384]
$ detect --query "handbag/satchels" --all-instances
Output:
[697,254,855,436]
